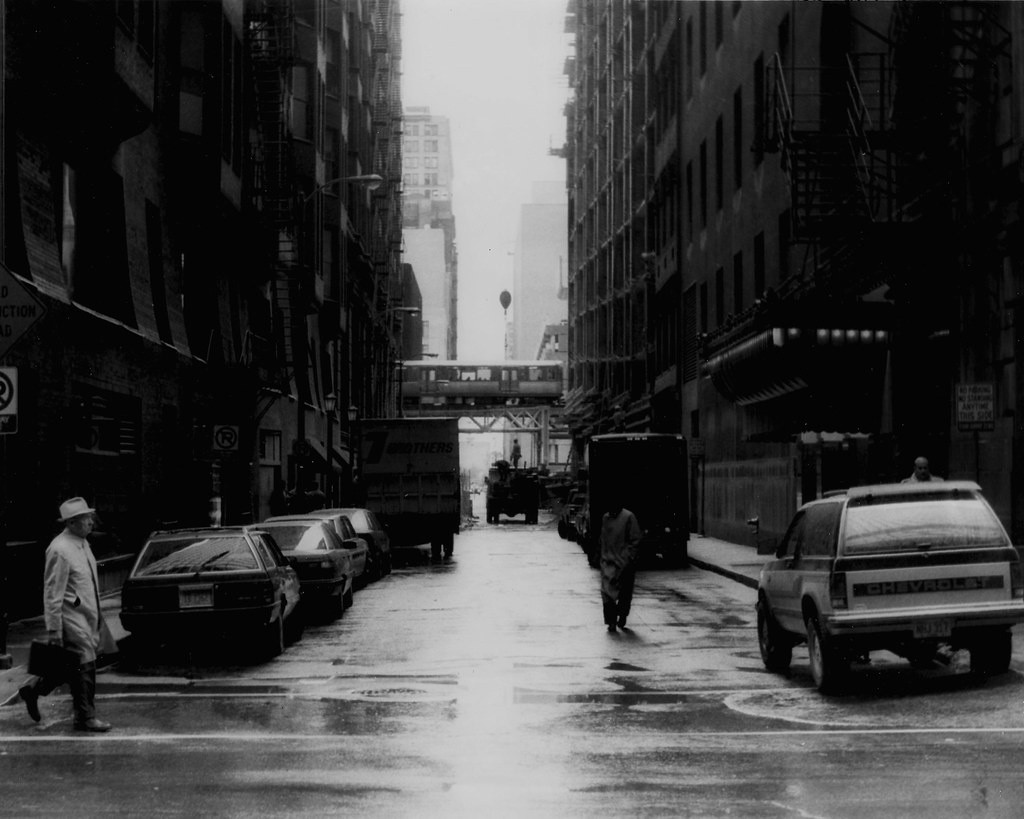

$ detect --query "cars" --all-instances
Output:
[752,480,1024,699]
[118,524,303,659]
[261,508,398,594]
[244,519,359,621]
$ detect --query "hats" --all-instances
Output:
[57,496,96,523]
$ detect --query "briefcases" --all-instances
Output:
[27,637,83,684]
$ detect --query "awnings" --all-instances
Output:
[296,437,357,473]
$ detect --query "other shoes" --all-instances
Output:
[617,616,626,628]
[608,623,616,632]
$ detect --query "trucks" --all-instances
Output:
[483,459,541,526]
[580,430,692,571]
[357,416,462,561]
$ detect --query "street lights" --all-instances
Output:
[324,391,339,509]
[346,404,358,508]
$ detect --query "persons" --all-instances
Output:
[901,456,943,485]
[510,439,522,472]
[268,478,326,517]
[600,490,641,632]
[18,496,112,731]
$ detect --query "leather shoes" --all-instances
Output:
[73,716,113,732]
[19,684,43,723]
